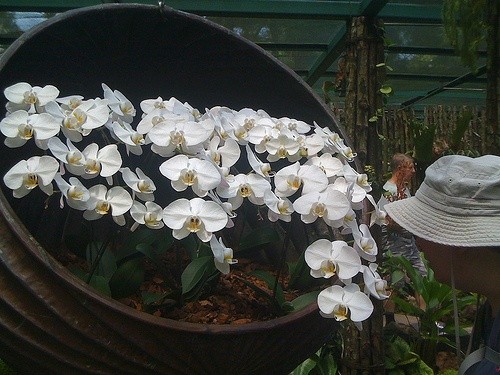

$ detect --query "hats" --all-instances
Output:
[383,154,500,249]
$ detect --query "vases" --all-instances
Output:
[1,0,370,375]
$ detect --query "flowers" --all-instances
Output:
[0,81,392,328]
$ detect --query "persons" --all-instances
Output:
[383,155,500,375]
[370,154,428,277]
